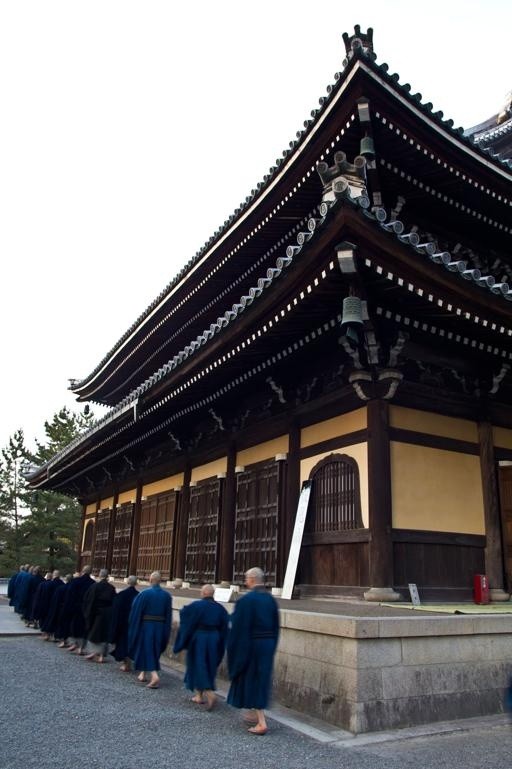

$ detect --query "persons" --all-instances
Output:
[108,575,140,672]
[7,563,96,655]
[127,571,172,689]
[173,584,228,712]
[225,566,281,734]
[81,568,116,663]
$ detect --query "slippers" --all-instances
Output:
[135,677,159,689]
[243,715,268,734]
[192,695,217,710]
